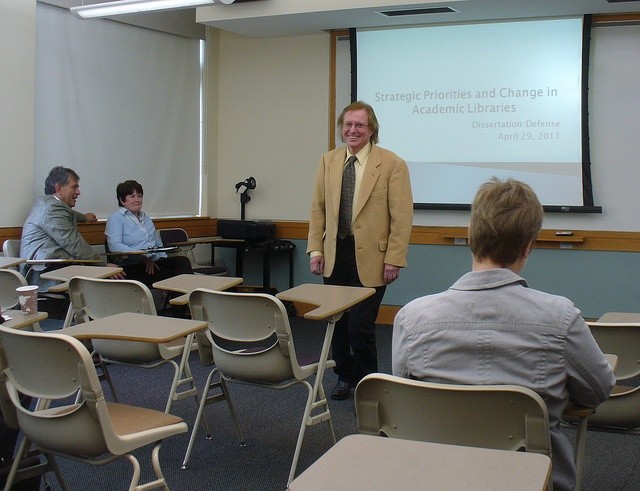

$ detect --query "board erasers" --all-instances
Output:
[555,232,573,236]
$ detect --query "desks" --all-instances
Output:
[561,353,618,491]
[283,434,553,491]
[272,284,377,362]
[209,237,296,295]
[0,310,49,333]
[40,266,124,328]
[33,313,208,412]
[597,312,640,324]
[27,259,103,266]
[0,257,26,269]
[152,273,244,307]
[99,245,182,256]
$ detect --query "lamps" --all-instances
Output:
[68,1,215,21]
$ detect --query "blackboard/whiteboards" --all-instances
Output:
[327,13,639,233]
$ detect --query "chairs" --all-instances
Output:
[352,373,553,460]
[181,288,339,488]
[0,269,28,312]
[1,324,189,491]
[2,240,21,273]
[67,276,212,441]
[157,228,230,277]
[557,320,640,467]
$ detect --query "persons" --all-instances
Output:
[306,102,413,402]
[392,179,615,490]
[19,167,126,297]
[104,181,194,301]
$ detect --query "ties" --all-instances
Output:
[338,156,357,241]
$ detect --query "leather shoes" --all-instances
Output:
[330,380,353,400]
